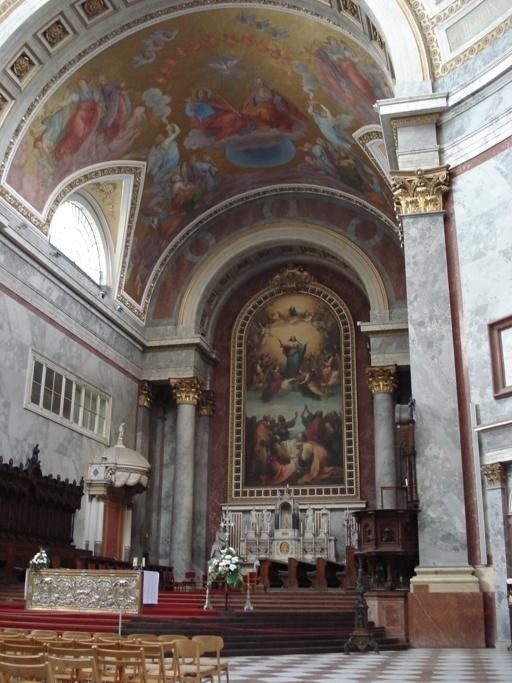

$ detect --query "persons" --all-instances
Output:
[246,304,341,484]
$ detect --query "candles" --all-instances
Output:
[299,520,315,535]
[256,521,270,534]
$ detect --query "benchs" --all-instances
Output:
[1,626,231,681]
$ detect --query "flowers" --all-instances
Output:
[206,541,248,592]
